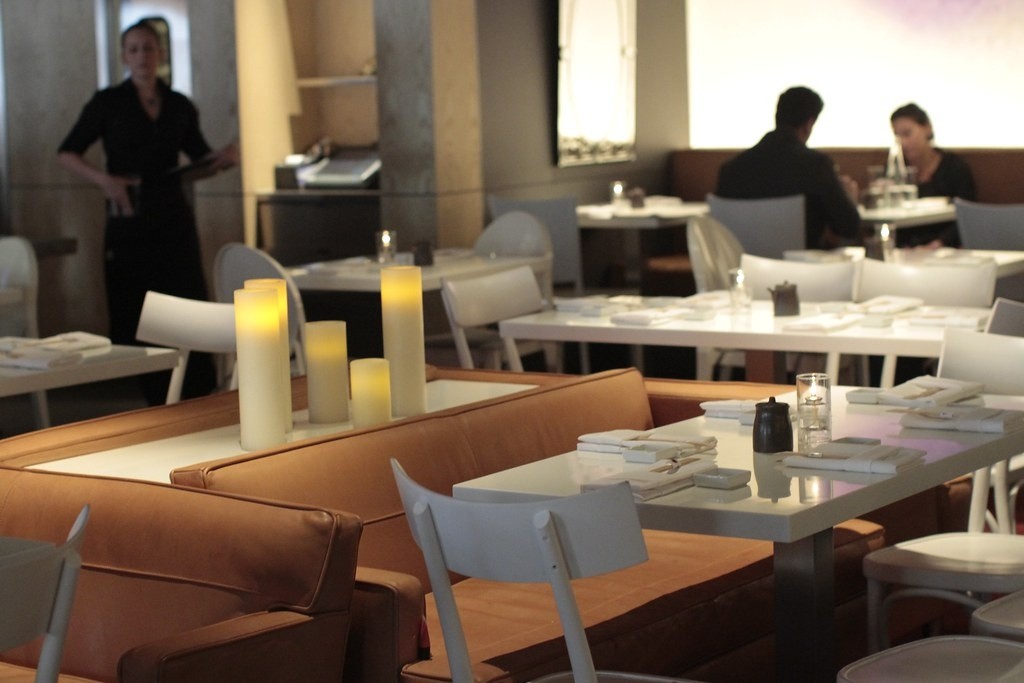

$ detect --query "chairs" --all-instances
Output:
[0,193,1022,682]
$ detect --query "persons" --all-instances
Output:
[713,87,874,255]
[55,23,237,407]
[882,104,975,253]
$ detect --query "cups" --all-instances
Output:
[874,225,896,260]
[609,180,626,209]
[375,231,397,264]
[796,373,831,457]
[728,266,751,313]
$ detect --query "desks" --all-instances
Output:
[290,246,550,298]
[24,379,542,486]
[576,199,707,290]
[857,190,957,251]
[452,381,1024,682]
[0,329,181,398]
[498,290,991,388]
[878,246,1024,283]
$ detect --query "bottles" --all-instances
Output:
[773,281,799,317]
[412,238,434,264]
[752,397,792,454]
